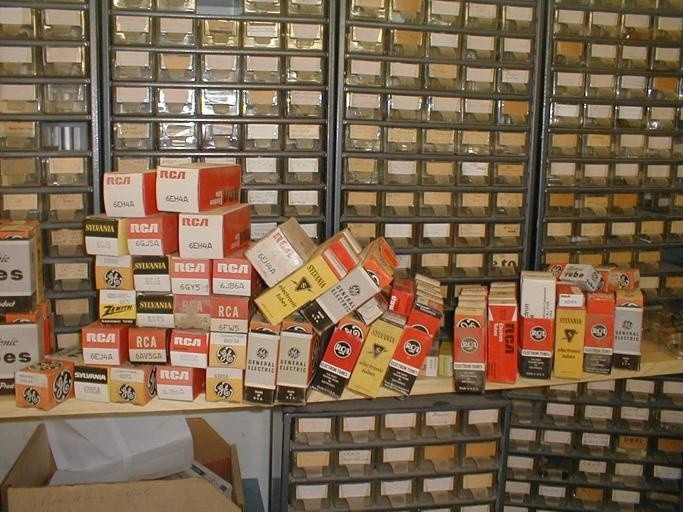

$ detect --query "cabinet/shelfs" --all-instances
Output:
[0,0,681,512]
